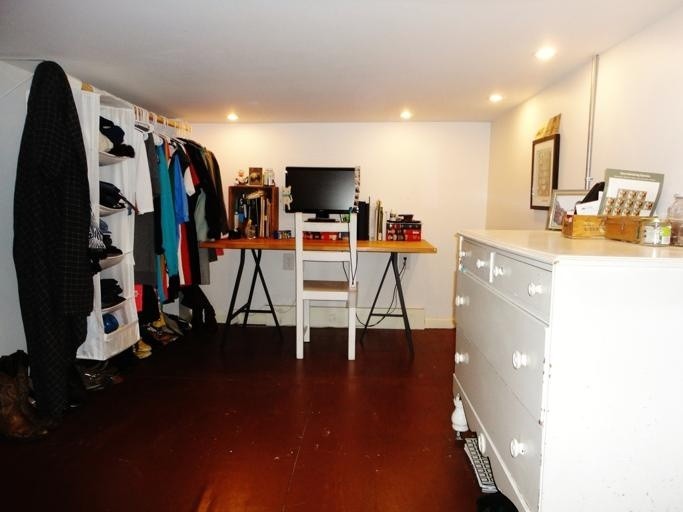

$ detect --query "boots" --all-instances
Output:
[0,351,58,440]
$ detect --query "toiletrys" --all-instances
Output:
[666,195,682,248]
[637,219,672,246]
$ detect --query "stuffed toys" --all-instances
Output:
[234,169,248,185]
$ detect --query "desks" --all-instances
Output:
[197,236,437,354]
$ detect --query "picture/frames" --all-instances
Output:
[545,188,592,232]
[529,133,561,210]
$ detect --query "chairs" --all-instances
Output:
[294,212,359,361]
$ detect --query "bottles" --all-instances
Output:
[262,168,275,187]
[390,208,396,221]
[639,194,683,247]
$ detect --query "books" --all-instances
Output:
[382,209,389,242]
[302,232,339,241]
[367,193,377,242]
[386,229,422,235]
[386,235,421,241]
[378,204,382,242]
[386,221,421,229]
[234,195,271,239]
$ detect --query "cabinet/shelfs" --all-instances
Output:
[451,233,683,511]
[75,87,143,362]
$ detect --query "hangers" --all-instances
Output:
[133,104,193,146]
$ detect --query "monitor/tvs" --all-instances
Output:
[284,166,355,221]
